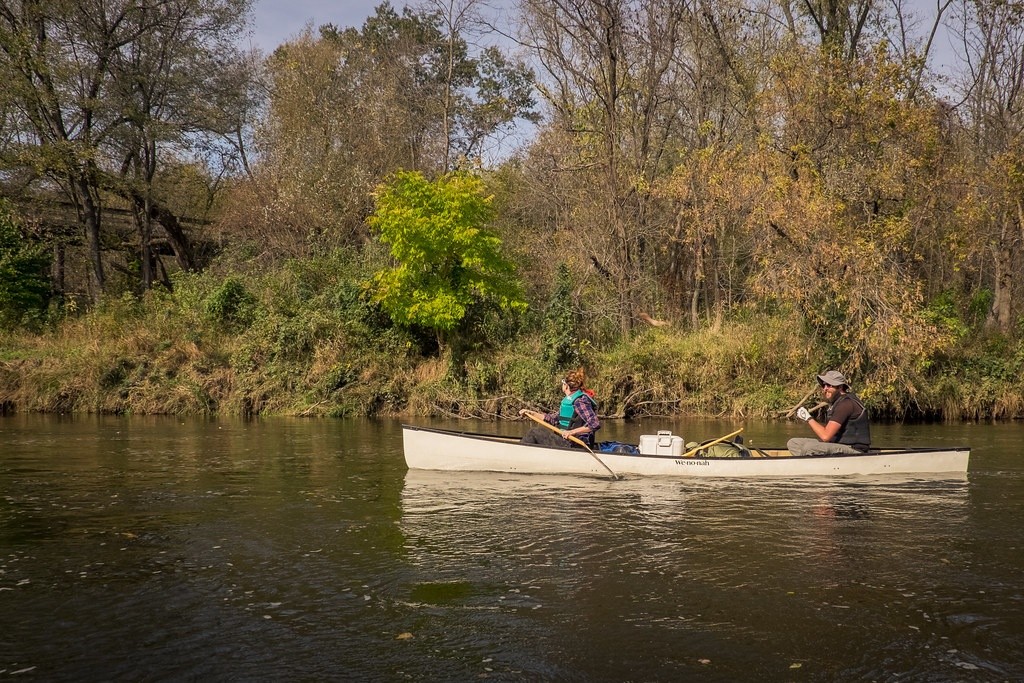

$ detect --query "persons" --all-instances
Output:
[787,370,871,456]
[518,370,602,450]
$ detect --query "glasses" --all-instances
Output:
[821,383,831,388]
[561,378,568,386]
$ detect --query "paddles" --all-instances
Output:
[524,412,620,480]
[683,426,745,457]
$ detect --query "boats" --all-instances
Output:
[402,422,973,475]
[400,474,971,514]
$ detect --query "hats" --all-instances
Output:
[817,371,852,391]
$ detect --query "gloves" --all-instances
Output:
[797,407,811,421]
